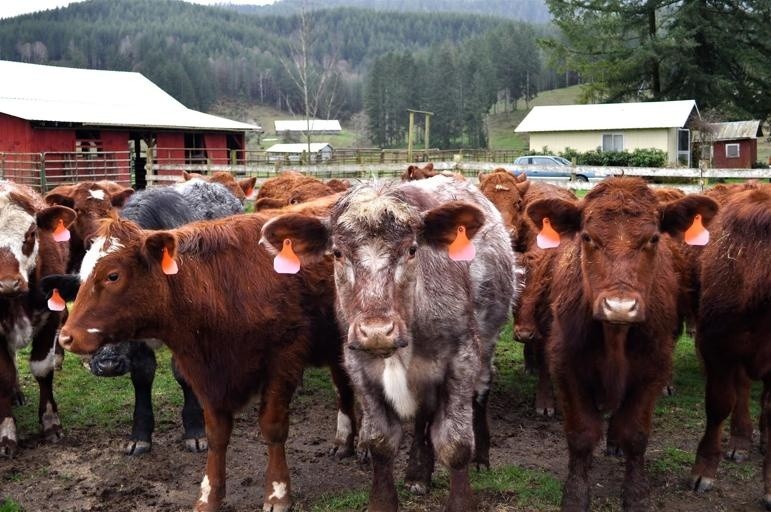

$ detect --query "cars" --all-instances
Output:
[510,156,613,183]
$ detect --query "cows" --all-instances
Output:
[0,161,771,511]
